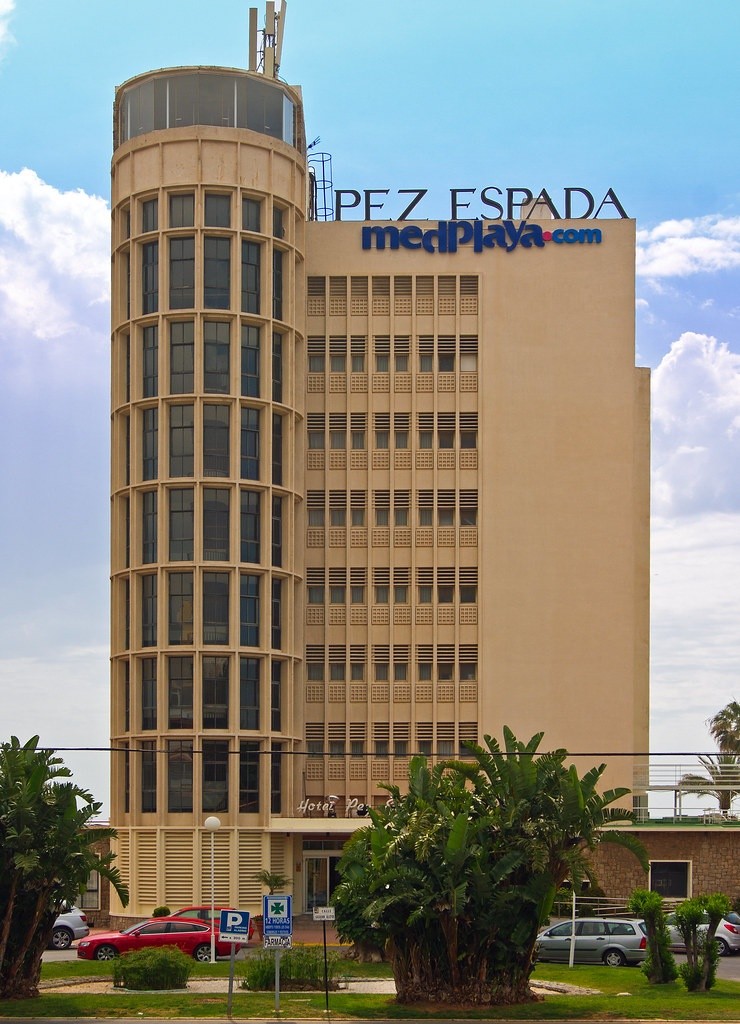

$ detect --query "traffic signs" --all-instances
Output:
[220,909,251,944]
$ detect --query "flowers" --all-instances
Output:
[254,914,263,922]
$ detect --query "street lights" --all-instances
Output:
[204,816,220,964]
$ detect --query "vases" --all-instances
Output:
[257,921,264,940]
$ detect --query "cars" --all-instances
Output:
[35,898,92,951]
[534,916,651,969]
[76,917,241,962]
[659,908,740,956]
[168,903,254,941]
